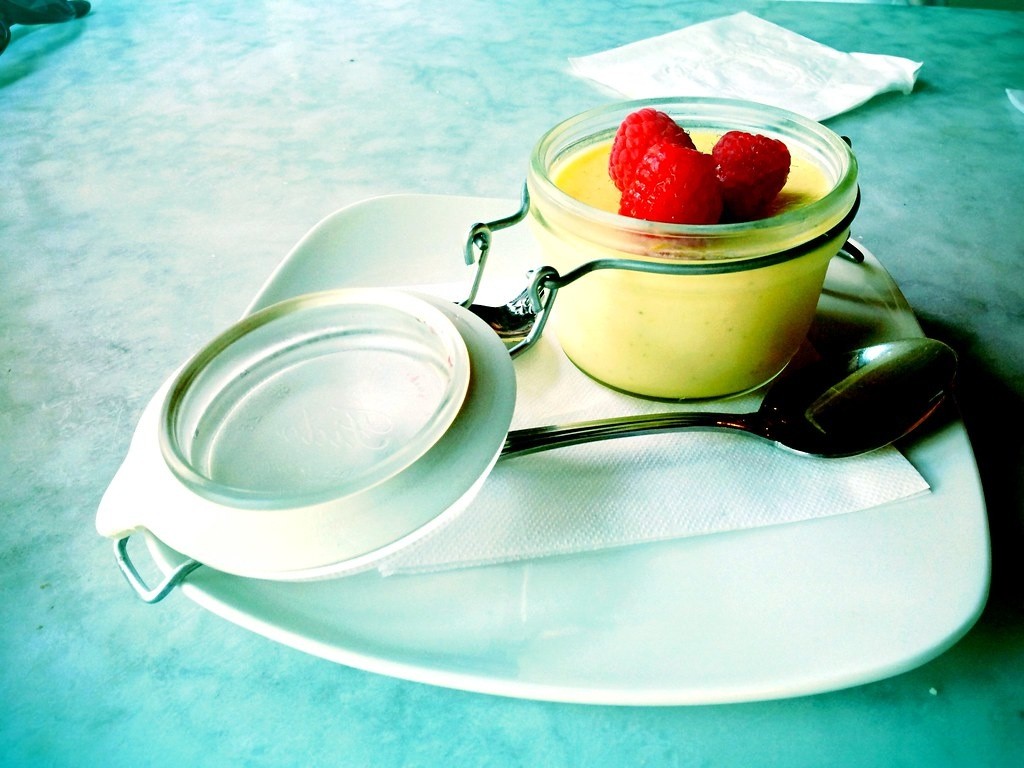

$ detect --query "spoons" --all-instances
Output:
[496,336,957,460]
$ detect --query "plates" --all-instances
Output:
[115,196,988,707]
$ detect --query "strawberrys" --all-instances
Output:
[608,108,696,193]
[716,132,791,212]
[618,142,723,245]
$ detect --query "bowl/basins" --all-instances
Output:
[98,97,858,605]
